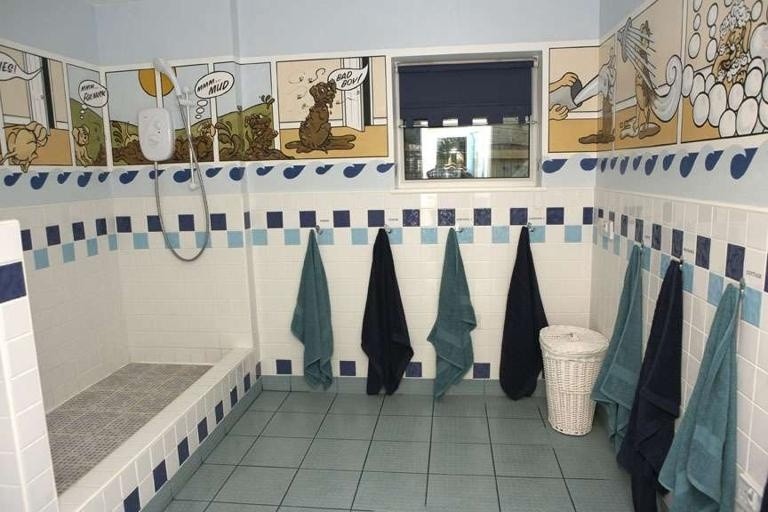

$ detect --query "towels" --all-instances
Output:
[590,244,644,464]
[617,260,684,510]
[657,282,742,511]
[499,226,549,402]
[426,228,476,401]
[291,229,335,392]
[359,228,415,397]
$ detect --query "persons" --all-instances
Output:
[549,70,576,122]
